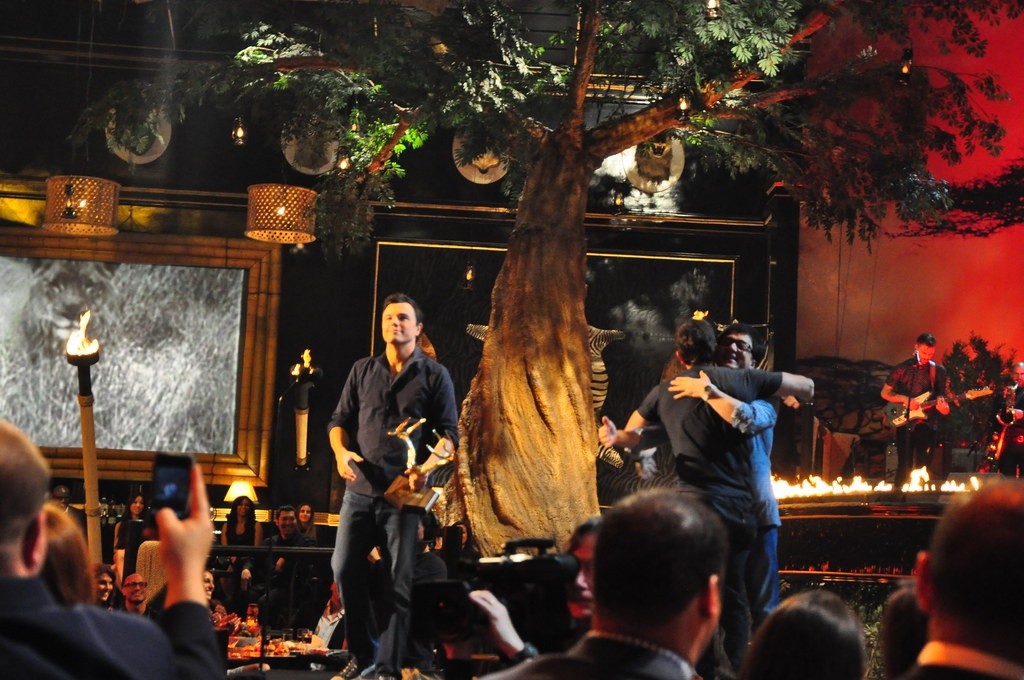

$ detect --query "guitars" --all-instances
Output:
[884,386,994,427]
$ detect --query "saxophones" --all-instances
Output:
[987,382,1020,464]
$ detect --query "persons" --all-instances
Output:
[240,503,317,625]
[0,418,228,680]
[599,320,814,674]
[367,511,484,674]
[880,333,951,491]
[879,477,1024,680]
[201,569,215,600]
[994,362,1024,479]
[220,496,263,601]
[113,492,154,591]
[328,294,459,680]
[93,563,158,622]
[468,487,728,680]
[52,485,88,546]
[738,590,867,680]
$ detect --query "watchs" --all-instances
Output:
[701,384,717,402]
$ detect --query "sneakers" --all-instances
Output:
[331,658,398,680]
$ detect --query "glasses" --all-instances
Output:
[719,336,753,353]
[123,581,147,588]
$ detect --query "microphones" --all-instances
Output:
[913,350,920,365]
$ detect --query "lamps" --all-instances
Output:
[224,480,259,504]
[243,184,318,244]
[42,175,120,237]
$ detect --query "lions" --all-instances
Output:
[19,256,128,360]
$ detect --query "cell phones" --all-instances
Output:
[141,452,197,530]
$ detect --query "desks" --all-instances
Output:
[227,652,352,680]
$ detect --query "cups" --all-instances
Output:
[282,628,312,653]
